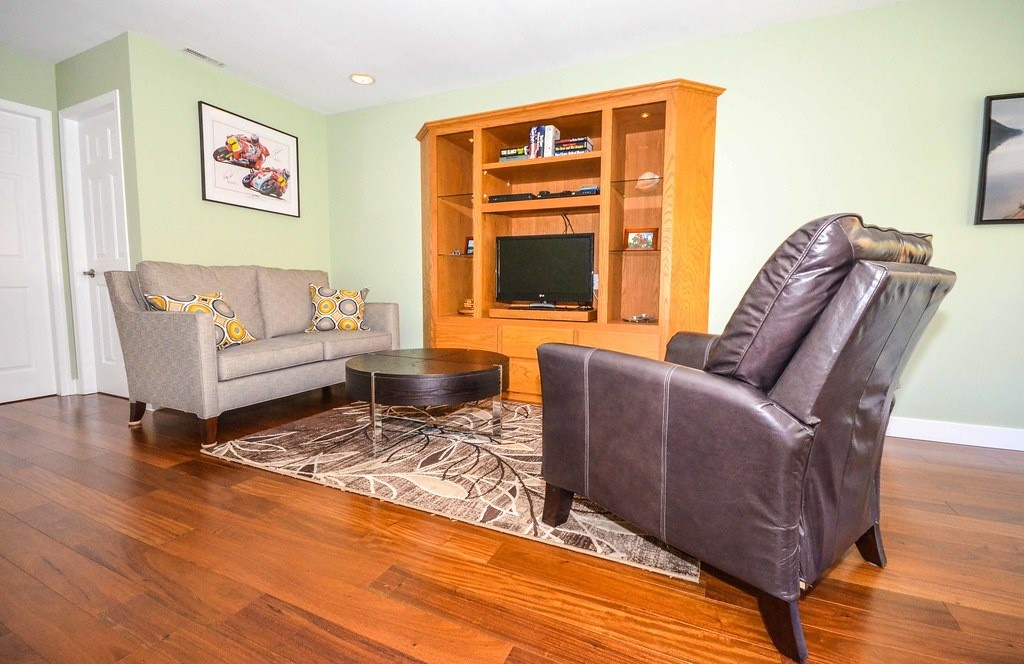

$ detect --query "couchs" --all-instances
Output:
[105,260,401,446]
[538,214,958,662]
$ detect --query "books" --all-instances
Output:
[499,125,593,162]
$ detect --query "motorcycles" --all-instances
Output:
[213,134,270,172]
[242,161,288,199]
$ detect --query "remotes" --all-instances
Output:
[529,303,555,308]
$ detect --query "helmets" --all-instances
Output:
[283,168,290,177]
[251,134,259,141]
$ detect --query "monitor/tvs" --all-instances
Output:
[496,233,595,309]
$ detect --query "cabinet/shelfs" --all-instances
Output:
[416,80,735,405]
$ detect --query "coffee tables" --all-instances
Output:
[347,346,508,446]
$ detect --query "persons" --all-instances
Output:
[236,133,263,170]
[253,167,290,198]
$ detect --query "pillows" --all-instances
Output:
[309,281,371,334]
[143,290,256,351]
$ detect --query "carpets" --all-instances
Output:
[198,398,710,585]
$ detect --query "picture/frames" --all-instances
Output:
[198,99,301,218]
[974,92,1024,224]
